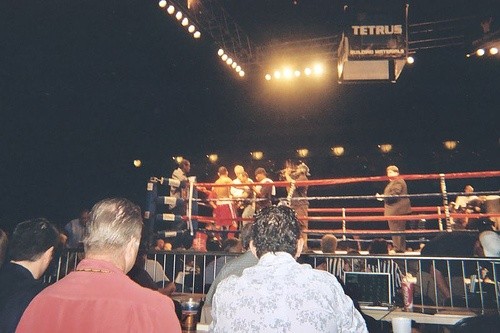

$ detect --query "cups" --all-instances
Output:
[180,297,200,333]
[392,318,411,333]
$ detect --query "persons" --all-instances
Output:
[15,197,181,333]
[281,164,309,253]
[0,218,55,333]
[377,166,410,253]
[254,168,274,206]
[238,172,257,227]
[40,209,499,300]
[450,183,486,219]
[231,165,252,199]
[170,159,209,232]
[212,206,371,333]
[209,166,239,243]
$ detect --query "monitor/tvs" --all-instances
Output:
[344,272,391,305]
